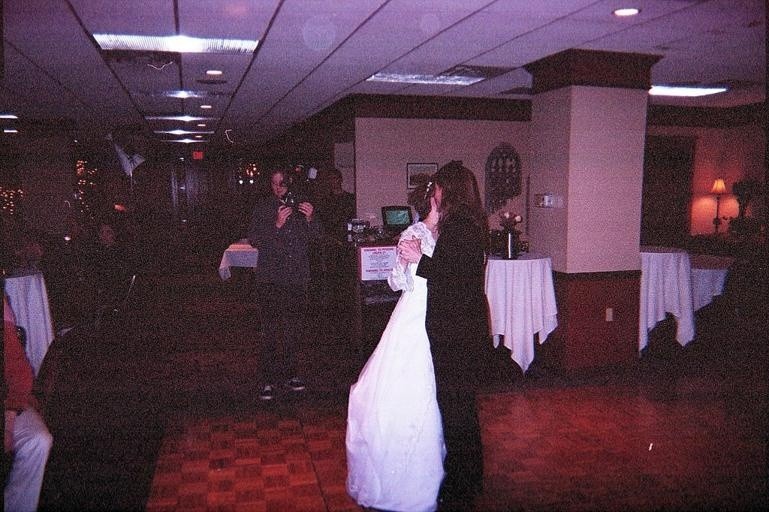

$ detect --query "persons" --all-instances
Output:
[249,168,352,399]
[344,172,442,512]
[0,214,132,511]
[399,162,490,512]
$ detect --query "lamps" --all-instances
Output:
[708,178,729,239]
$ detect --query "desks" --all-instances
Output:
[5,268,57,376]
[217,234,733,385]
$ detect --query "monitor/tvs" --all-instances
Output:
[381,205,414,228]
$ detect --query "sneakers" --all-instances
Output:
[258,381,275,402]
[282,377,306,391]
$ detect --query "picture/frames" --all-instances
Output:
[407,163,438,189]
